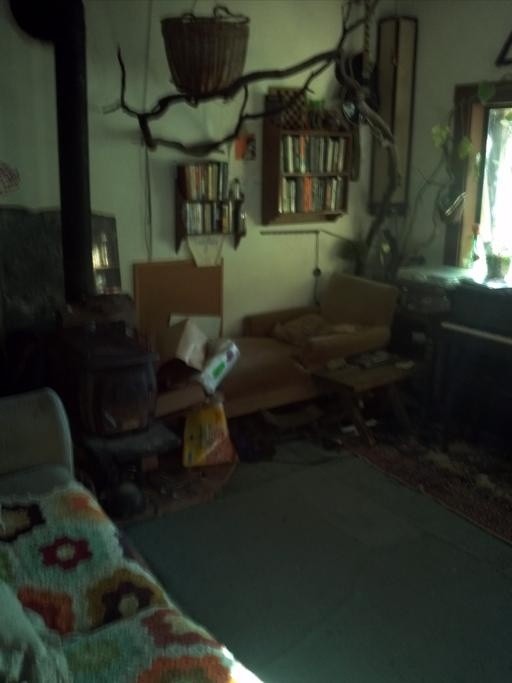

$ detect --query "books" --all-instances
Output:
[182,161,236,235]
[278,135,347,215]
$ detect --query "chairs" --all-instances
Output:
[206,273,400,431]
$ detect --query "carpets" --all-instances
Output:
[318,419,510,545]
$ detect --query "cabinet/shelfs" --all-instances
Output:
[262,93,353,226]
[174,192,246,254]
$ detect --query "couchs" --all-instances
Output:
[2,387,270,682]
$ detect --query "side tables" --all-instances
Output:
[312,350,413,447]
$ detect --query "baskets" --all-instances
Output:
[160,6,250,102]
